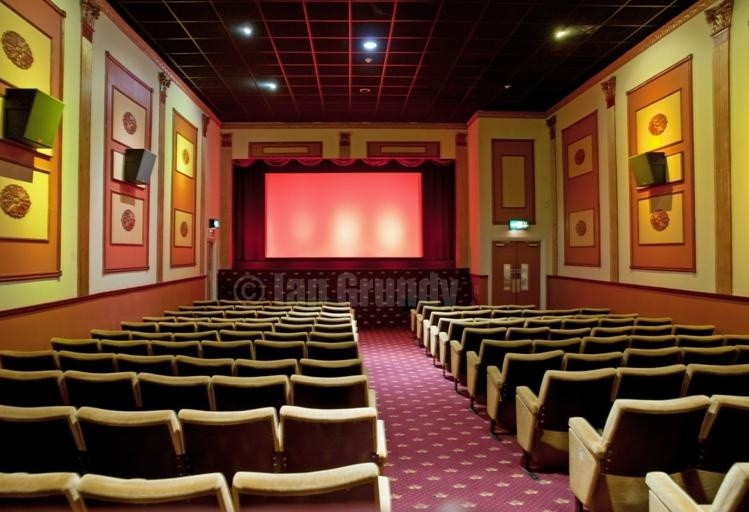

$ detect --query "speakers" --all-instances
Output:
[628,152,667,185]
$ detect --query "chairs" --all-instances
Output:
[682,363,747,400]
[77,472,235,511]
[515,368,618,480]
[2,472,83,510]
[568,395,712,510]
[644,463,748,511]
[693,394,748,460]
[611,363,687,402]
[76,406,184,481]
[177,406,280,485]
[2,299,376,415]
[278,404,387,474]
[230,461,393,512]
[1,405,88,476]
[406,298,747,441]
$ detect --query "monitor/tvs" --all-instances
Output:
[508,217,529,230]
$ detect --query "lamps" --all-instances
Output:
[207,217,220,231]
[625,149,667,188]
